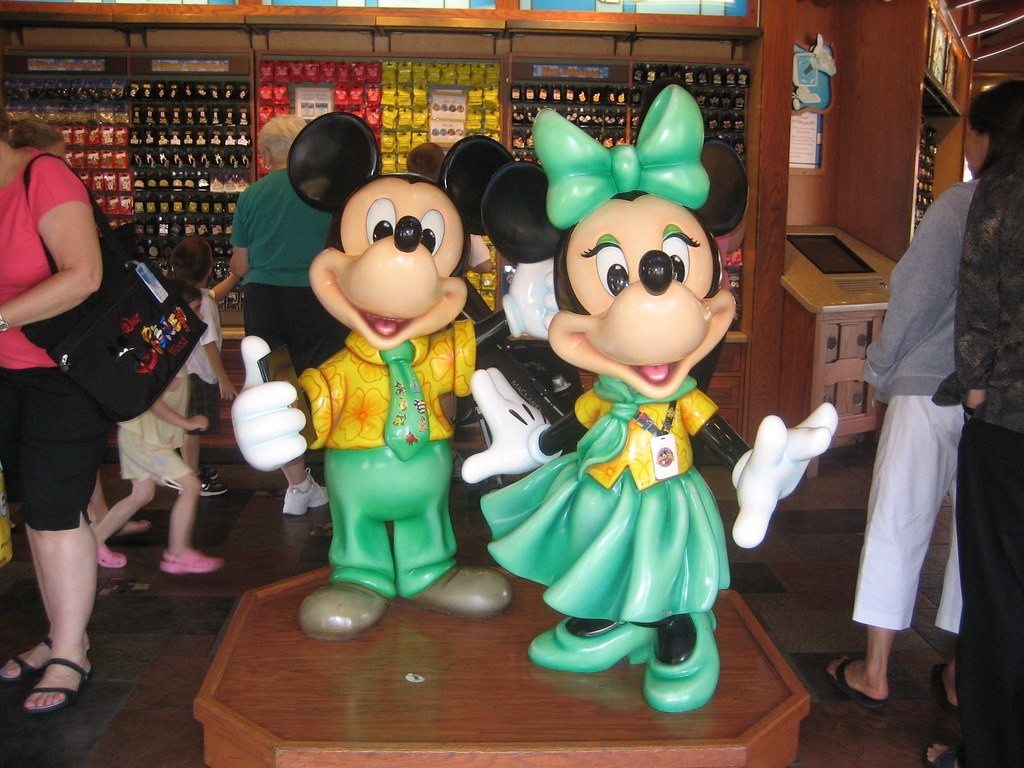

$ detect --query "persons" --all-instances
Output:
[822,80,1024,768]
[229,114,328,516]
[170,234,240,496]
[90,279,225,573]
[0,80,103,713]
[405,141,495,274]
[86,465,153,534]
[690,215,747,469]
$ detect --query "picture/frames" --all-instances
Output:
[944,44,958,100]
[928,14,948,86]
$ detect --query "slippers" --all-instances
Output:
[818,652,884,708]
[935,663,958,713]
[0,632,90,681]
[24,657,91,715]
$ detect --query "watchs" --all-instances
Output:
[0,315,9,331]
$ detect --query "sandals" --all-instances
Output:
[158,549,224,573]
[95,543,126,568]
[921,741,961,768]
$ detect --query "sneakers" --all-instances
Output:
[195,466,219,478]
[282,470,330,516]
[179,479,227,495]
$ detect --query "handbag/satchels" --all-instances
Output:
[21,151,207,423]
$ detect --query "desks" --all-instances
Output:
[192,566,811,768]
